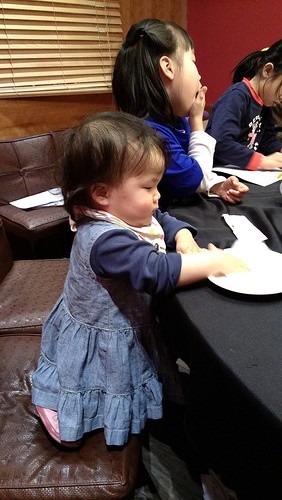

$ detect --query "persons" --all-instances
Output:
[204,38,282,171]
[112,20,249,205]
[28,111,250,485]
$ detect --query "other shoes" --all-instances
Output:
[25,374,83,448]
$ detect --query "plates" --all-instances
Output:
[206,248,281,295]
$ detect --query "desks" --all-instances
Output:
[166,173,282,422]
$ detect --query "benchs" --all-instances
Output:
[0,127,142,500]
